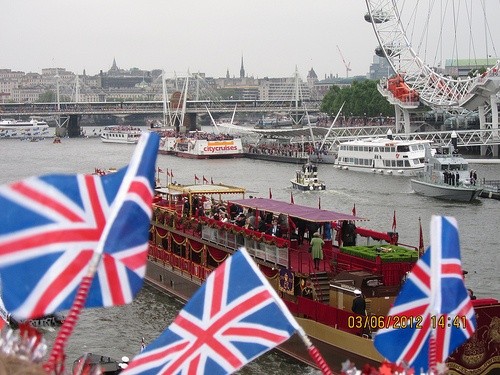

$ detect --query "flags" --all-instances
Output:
[370,215,476,372]
[0,128,161,312]
[120,248,298,375]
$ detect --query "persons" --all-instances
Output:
[352,290,366,315]
[0,120,489,323]
[310,232,325,270]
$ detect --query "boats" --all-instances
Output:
[0,118,55,139]
[243,137,336,164]
[100,129,141,144]
[290,153,326,191]
[150,129,244,160]
[72,351,125,375]
[84,162,500,374]
[332,128,438,176]
[412,131,500,202]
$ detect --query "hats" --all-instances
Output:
[354,289,362,295]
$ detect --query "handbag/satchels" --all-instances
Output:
[308,246,312,253]
